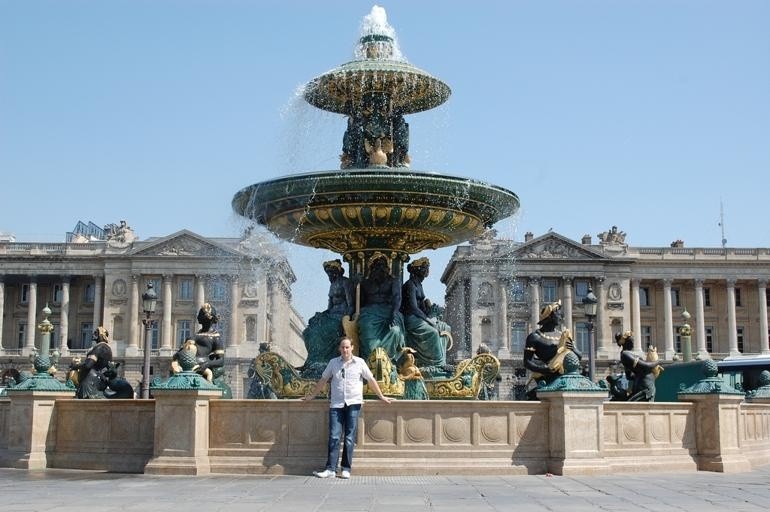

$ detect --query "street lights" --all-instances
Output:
[581,284,598,385]
[579,361,589,378]
[0,375,9,386]
[222,373,232,387]
[506,373,521,400]
[138,281,161,401]
[613,360,624,377]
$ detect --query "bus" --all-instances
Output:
[717,352,770,393]
[612,370,628,391]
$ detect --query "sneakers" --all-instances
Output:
[315,470,335,478]
[341,471,350,479]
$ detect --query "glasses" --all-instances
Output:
[341,368,345,379]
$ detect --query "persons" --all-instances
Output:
[171,302,224,376]
[358,252,405,359]
[299,259,354,378]
[523,302,582,398]
[399,256,451,368]
[618,330,663,402]
[248,342,278,399]
[70,327,112,398]
[301,337,395,479]
